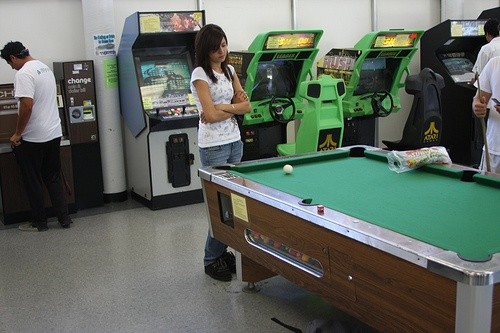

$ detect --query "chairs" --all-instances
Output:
[277,75,347,156]
[382,67,446,151]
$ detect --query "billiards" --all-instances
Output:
[283,165,293,174]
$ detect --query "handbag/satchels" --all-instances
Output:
[386,146,450,173]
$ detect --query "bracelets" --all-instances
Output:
[232,104,235,113]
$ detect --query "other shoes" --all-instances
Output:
[58,217,72,228]
[205,259,230,281]
[221,251,237,274]
[18,223,41,231]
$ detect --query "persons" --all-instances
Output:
[190,24,252,282]
[473,56,500,174]
[471,17,500,76]
[0,41,73,232]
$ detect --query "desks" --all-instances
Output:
[198,145,500,333]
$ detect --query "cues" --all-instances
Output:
[476,70,492,173]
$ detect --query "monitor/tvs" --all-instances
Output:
[441,56,475,83]
[352,58,404,97]
[250,59,305,102]
[137,51,194,110]
